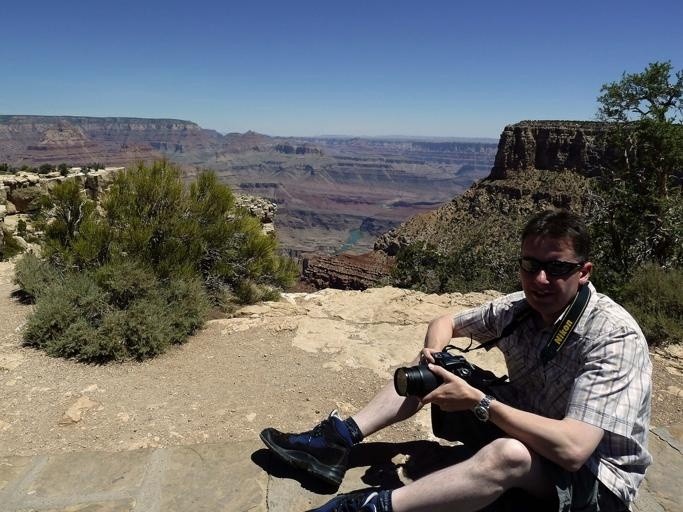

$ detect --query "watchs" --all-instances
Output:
[472,394,494,423]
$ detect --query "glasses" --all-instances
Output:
[517,256,585,277]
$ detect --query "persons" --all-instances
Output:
[257,211,654,512]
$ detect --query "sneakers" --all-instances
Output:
[305,487,380,512]
[260,409,351,484]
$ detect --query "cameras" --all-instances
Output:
[394,351,478,397]
